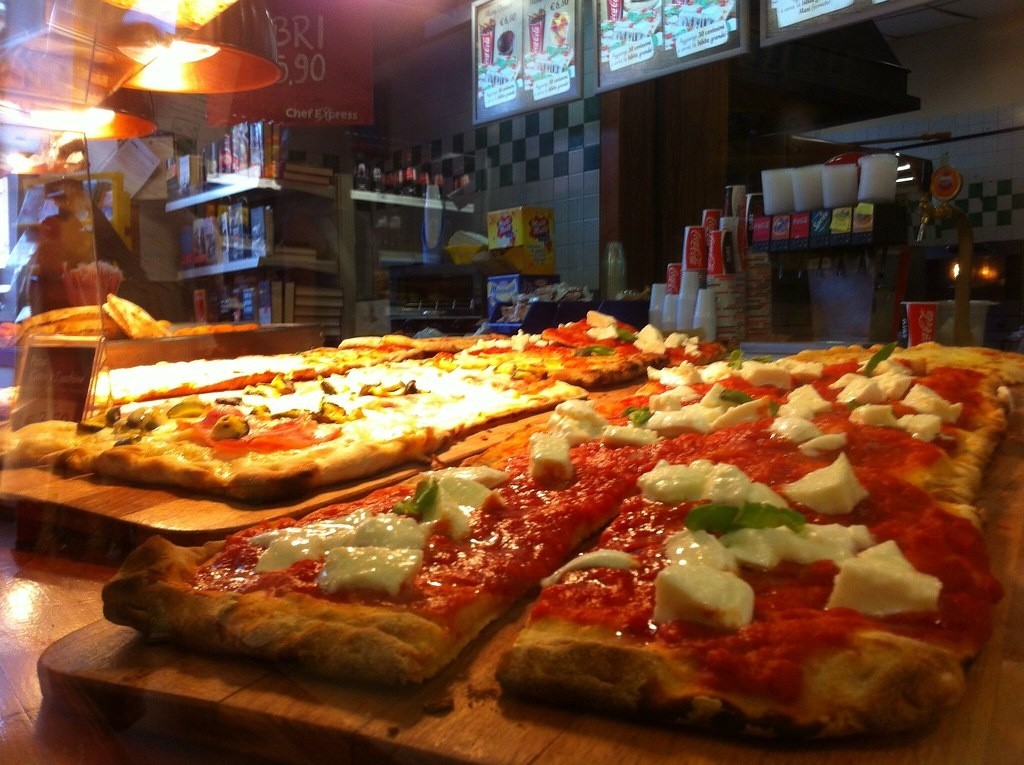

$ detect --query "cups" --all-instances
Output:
[549,22,569,46]
[649,185,772,341]
[607,0,624,20]
[529,18,545,52]
[480,29,495,65]
[901,301,938,347]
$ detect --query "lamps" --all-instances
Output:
[0,0,291,140]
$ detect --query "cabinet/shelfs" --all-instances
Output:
[351,176,485,338]
[166,174,351,343]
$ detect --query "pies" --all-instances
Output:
[0,291,1024,743]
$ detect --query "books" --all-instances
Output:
[164,119,343,336]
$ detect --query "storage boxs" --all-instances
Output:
[178,120,290,201]
[487,204,560,324]
[194,274,257,323]
[180,204,274,269]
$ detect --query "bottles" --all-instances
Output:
[383,149,445,198]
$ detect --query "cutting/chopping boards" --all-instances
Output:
[36,383,1024,765]
[0,397,557,543]
